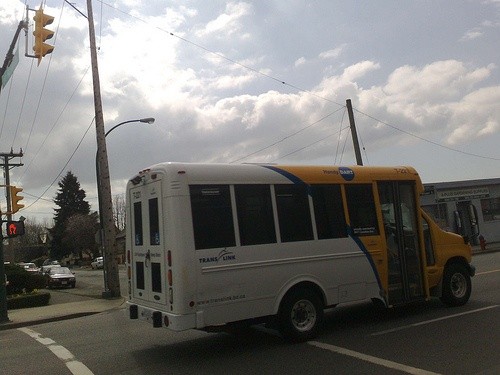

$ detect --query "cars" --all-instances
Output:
[91,257,103,269]
[16,258,76,289]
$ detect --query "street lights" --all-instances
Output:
[96,118,156,299]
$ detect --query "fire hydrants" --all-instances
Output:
[479,235,487,250]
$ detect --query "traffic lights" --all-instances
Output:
[10,186,25,215]
[32,9,55,66]
[6,221,24,236]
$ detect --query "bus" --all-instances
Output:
[127,162,476,343]
